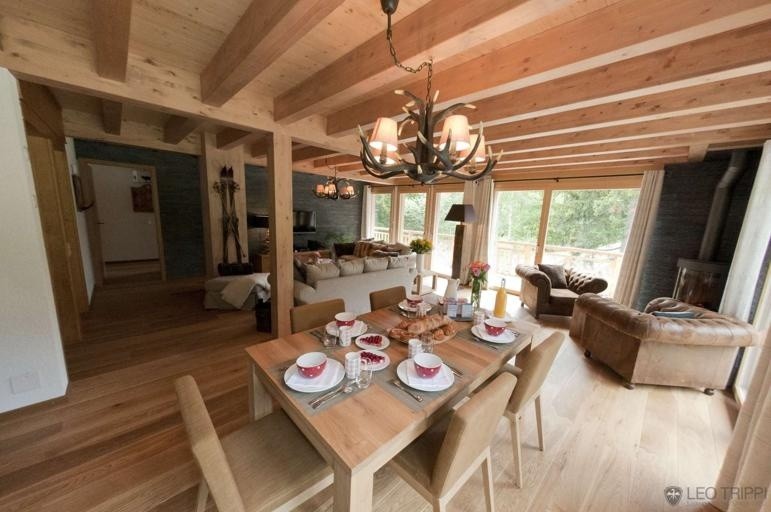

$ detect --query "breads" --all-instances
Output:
[398,315,454,337]
[422,333,431,342]
[401,333,415,341]
[389,329,405,338]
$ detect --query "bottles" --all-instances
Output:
[471,275,480,311]
[405,268,421,298]
[494,279,507,318]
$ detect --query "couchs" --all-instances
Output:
[204,273,271,311]
[516,265,607,320]
[570,293,758,395]
[268,268,418,317]
[330,247,417,268]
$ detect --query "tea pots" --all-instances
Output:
[444,278,461,303]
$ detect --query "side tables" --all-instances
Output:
[415,271,438,296]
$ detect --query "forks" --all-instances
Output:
[387,378,424,402]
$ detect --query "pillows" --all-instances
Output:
[358,242,364,257]
[654,312,695,317]
[369,250,382,256]
[387,245,401,252]
[334,242,355,257]
[353,238,374,255]
[364,241,383,255]
[538,264,567,288]
[342,255,359,261]
[364,257,389,272]
[373,251,399,257]
[388,256,408,268]
[303,263,340,287]
[371,243,388,250]
[358,241,363,257]
[339,256,368,275]
[400,247,412,255]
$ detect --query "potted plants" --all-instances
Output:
[212,165,254,276]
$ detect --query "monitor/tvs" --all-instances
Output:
[293,208,318,234]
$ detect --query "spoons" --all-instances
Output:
[312,385,358,409]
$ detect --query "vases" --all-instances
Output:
[471,280,480,312]
[416,255,424,274]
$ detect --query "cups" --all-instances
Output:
[473,311,484,327]
[344,352,361,380]
[354,357,373,389]
[408,339,422,358]
[322,327,337,350]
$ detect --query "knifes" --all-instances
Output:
[309,377,365,406]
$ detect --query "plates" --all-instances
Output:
[355,333,391,351]
[396,357,455,392]
[358,349,391,372]
[283,358,346,393]
[386,316,460,345]
[398,300,432,313]
[325,320,368,338]
[471,324,516,344]
[486,310,513,322]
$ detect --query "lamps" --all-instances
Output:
[445,204,477,224]
[312,177,360,201]
[358,89,504,184]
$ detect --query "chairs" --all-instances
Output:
[389,372,517,512]
[290,299,345,334]
[369,286,406,311]
[173,375,334,512]
[468,331,565,489]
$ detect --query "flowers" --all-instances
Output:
[467,262,490,285]
[409,239,433,254]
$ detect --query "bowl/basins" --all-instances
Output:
[295,351,327,379]
[406,294,423,309]
[335,312,356,329]
[484,319,507,336]
[413,352,443,380]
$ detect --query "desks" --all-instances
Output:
[258,250,331,272]
